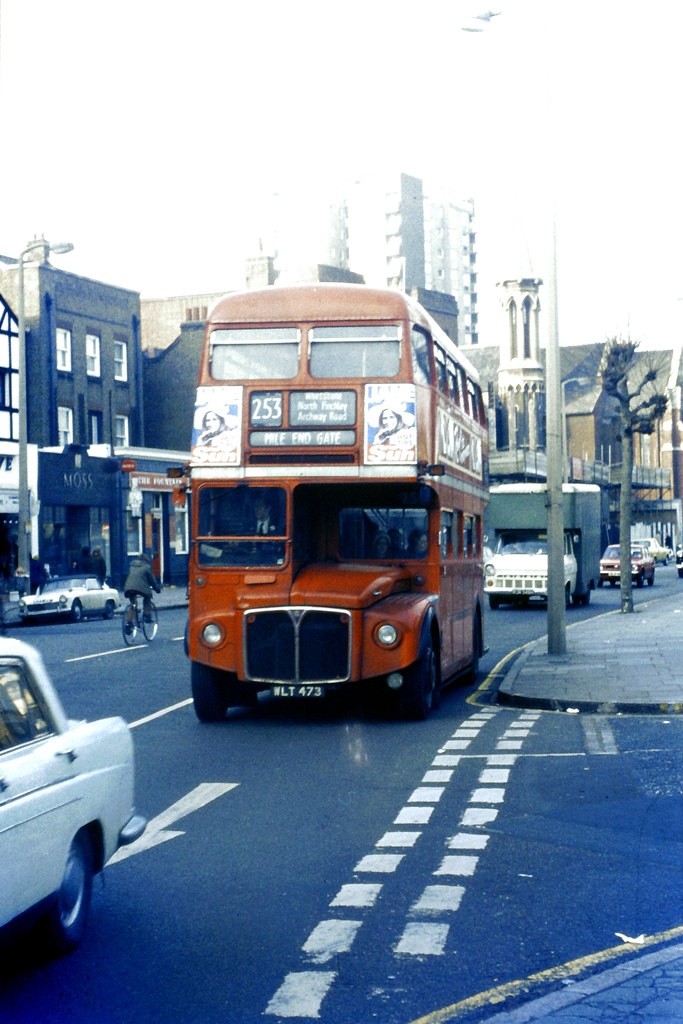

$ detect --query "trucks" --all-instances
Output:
[482,483,601,610]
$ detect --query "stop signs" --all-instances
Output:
[121,460,137,473]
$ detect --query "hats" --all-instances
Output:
[193,401,239,431]
[372,529,392,549]
[366,397,414,429]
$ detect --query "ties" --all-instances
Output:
[256,522,265,551]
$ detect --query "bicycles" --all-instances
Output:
[122,586,158,646]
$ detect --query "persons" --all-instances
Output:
[197,407,237,453]
[124,553,161,635]
[241,498,283,552]
[385,528,428,559]
[78,546,106,585]
[373,408,414,451]
[30,553,48,595]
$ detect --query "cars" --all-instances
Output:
[632,538,670,567]
[0,637,147,960]
[598,544,656,589]
[17,574,121,627]
[676,543,683,579]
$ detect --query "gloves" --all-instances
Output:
[155,588,161,594]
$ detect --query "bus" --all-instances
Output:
[167,282,490,724]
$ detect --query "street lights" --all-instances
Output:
[16,241,76,595]
[561,375,593,485]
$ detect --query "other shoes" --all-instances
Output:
[144,617,154,623]
[125,621,133,636]
